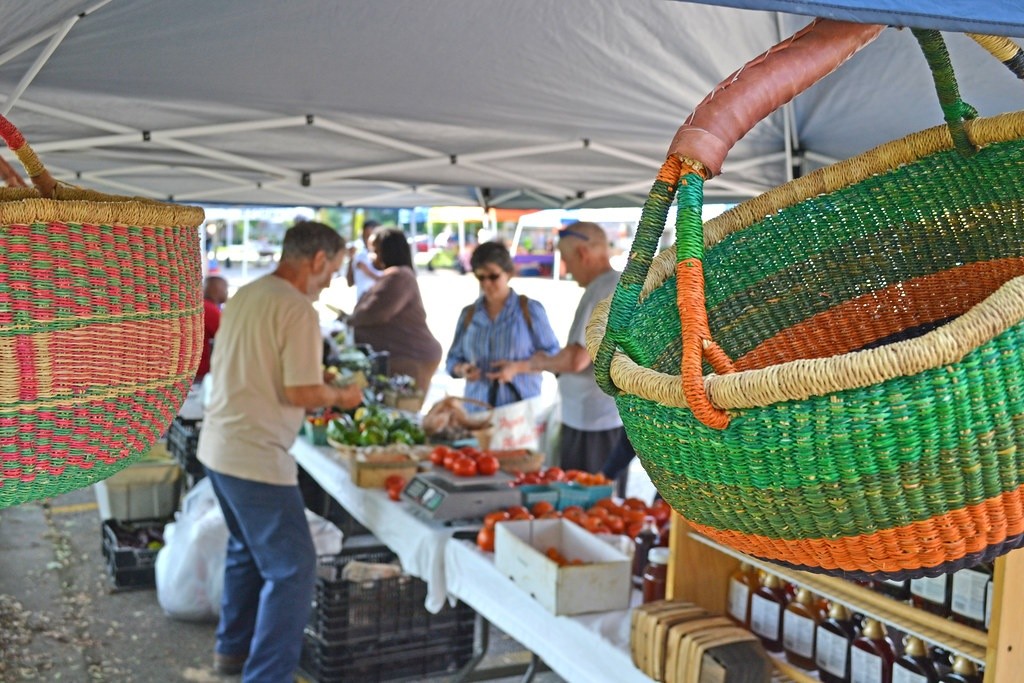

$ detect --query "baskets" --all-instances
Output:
[584,18,1023,581]
[0,119,202,511]
[423,395,495,455]
[98,518,172,589]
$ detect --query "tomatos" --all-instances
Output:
[306,407,343,425]
[431,442,612,487]
[479,498,673,566]
[384,474,407,500]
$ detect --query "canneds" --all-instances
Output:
[642,546,671,603]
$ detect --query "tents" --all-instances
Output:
[398,206,639,280]
[0,0,1024,210]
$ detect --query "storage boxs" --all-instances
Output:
[101,517,171,591]
[165,414,204,474]
[489,517,634,617]
[299,545,476,682]
[94,457,182,523]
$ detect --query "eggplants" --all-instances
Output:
[319,329,416,394]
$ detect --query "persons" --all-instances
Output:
[445,242,561,413]
[346,219,382,299]
[531,221,636,499]
[337,227,442,409]
[192,276,228,382]
[196,220,361,683]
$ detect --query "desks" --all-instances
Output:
[292,432,658,682]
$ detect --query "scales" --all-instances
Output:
[399,464,523,527]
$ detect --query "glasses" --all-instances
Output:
[559,229,588,240]
[476,267,503,281]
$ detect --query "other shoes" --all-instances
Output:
[212,651,248,673]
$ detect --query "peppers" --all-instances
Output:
[330,409,426,445]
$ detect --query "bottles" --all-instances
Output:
[632,515,659,591]
[644,545,668,606]
[726,561,996,683]
